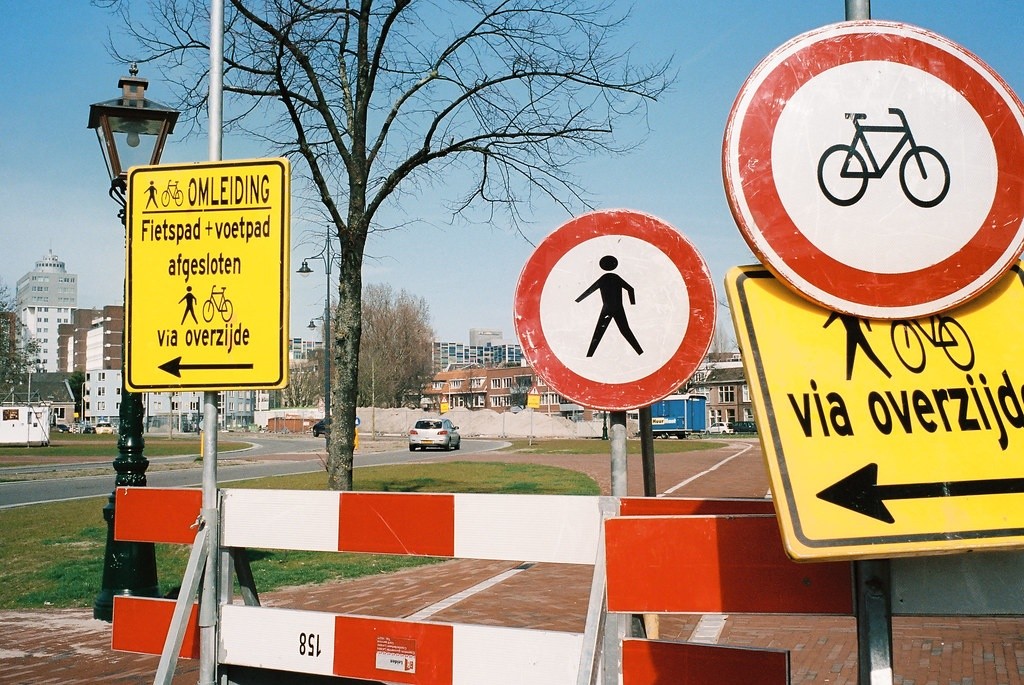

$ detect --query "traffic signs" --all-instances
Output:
[720,262,1024,563]
[123,162,287,393]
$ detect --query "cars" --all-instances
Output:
[312,415,333,437]
[96,423,113,435]
[83,427,96,435]
[704,422,734,435]
[408,418,462,451]
[56,424,72,433]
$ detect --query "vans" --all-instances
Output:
[733,421,758,435]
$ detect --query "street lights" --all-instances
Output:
[91,61,180,621]
[296,225,335,453]
[304,299,333,455]
